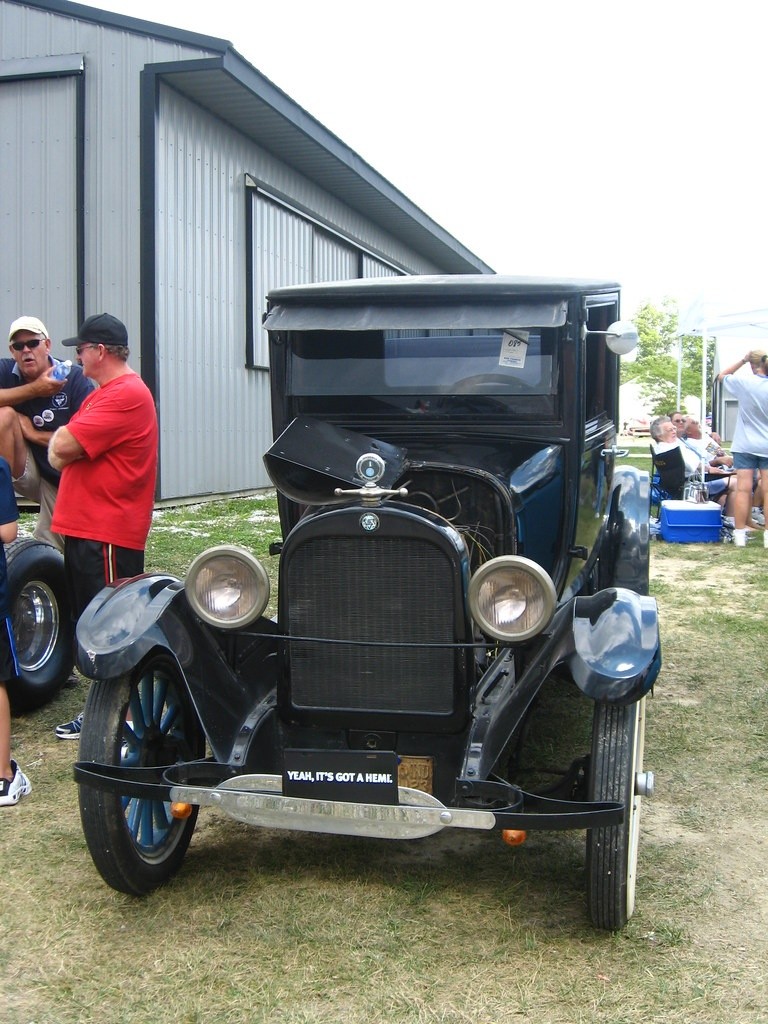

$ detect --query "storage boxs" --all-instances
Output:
[660,500,722,544]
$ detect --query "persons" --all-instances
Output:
[650,412,768,540]
[718,351,768,548]
[49,313,157,739]
[0,316,94,684]
[0,458,32,806]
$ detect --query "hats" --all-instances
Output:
[61,312,128,346]
[7,315,50,341]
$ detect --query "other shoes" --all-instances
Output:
[723,516,749,533]
[65,671,80,688]
[751,506,765,526]
[732,529,747,547]
[762,530,768,549]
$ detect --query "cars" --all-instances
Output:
[72,272,663,932]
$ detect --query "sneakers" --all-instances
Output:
[55,711,86,740]
[0,759,32,807]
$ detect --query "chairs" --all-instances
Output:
[649,444,738,536]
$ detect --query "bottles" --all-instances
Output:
[48,359,72,379]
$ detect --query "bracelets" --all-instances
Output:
[742,359,746,364]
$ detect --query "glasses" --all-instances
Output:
[75,346,96,354]
[691,420,699,424]
[11,337,45,351]
[673,418,686,423]
